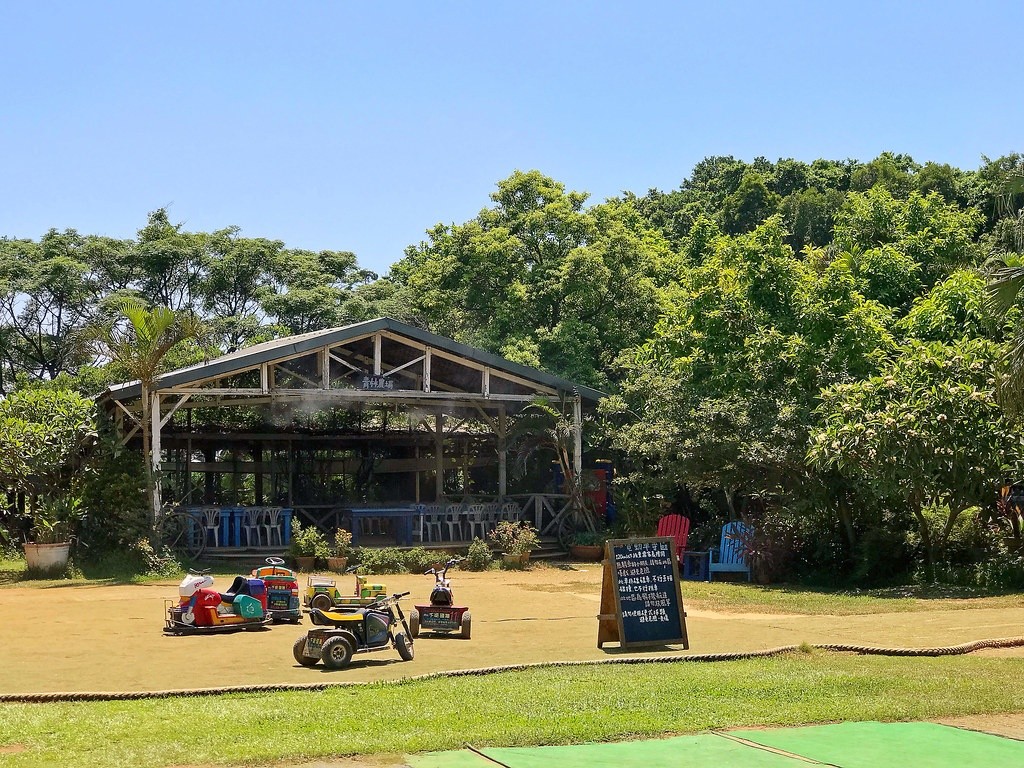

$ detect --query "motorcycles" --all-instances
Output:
[410,558,472,638]
[293,592,415,670]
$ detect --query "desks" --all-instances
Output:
[681,551,709,582]
[186,507,232,548]
[352,509,416,546]
[412,510,520,543]
[232,507,293,548]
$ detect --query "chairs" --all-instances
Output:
[464,503,489,542]
[657,514,689,574]
[197,504,221,547]
[423,505,443,542]
[260,506,282,546]
[341,502,424,536]
[442,504,463,542]
[499,502,522,523]
[240,506,265,549]
[479,503,501,543]
[709,522,755,583]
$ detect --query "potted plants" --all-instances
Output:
[357,535,495,575]
[567,532,605,561]
[512,520,542,564]
[325,527,353,571]
[486,520,529,567]
[22,493,89,576]
[289,516,331,573]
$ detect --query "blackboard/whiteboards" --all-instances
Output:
[604,536,689,653]
